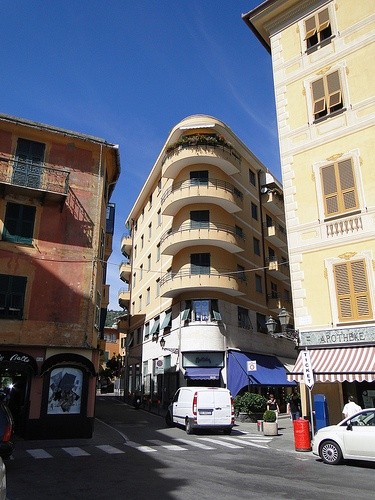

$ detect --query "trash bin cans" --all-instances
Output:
[293,419,310,453]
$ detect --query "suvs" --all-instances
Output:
[0,401,16,457]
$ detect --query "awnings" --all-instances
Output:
[226,352,298,398]
[184,368,220,382]
[286,348,375,382]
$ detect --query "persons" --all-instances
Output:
[342,396,362,425]
[266,393,280,424]
[125,390,161,412]
[0,392,12,499]
[287,392,301,420]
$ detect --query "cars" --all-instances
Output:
[311,408,375,463]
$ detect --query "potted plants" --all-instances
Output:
[263,411,278,436]
[239,392,268,423]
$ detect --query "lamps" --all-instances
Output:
[160,337,179,357]
[278,307,301,344]
[266,315,296,343]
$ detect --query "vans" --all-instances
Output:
[167,386,235,436]
